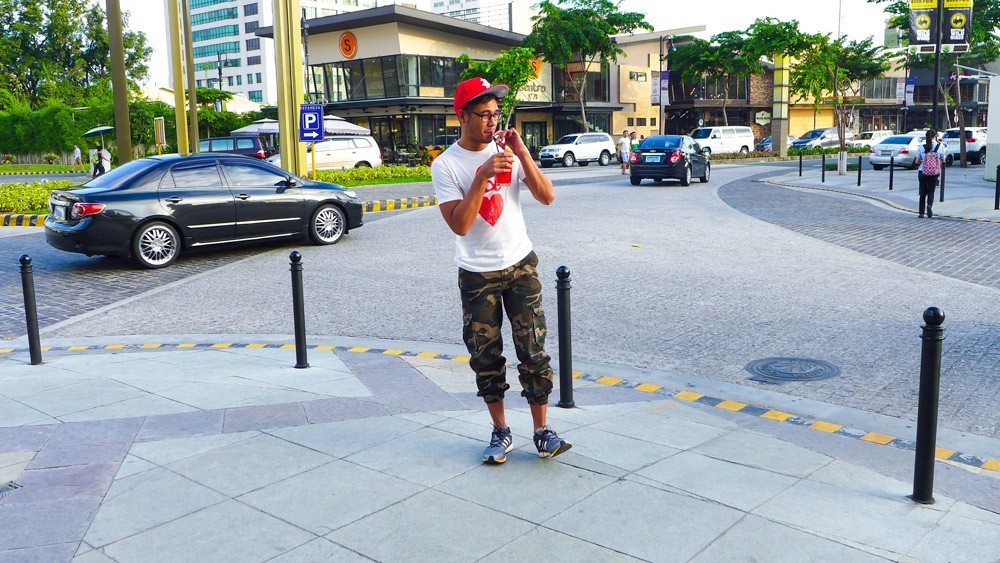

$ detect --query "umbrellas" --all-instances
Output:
[82,125,116,148]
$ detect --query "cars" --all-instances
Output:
[44,153,364,269]
[630,134,711,186]
[869,130,955,170]
[756,135,796,154]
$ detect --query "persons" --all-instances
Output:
[431,76,572,463]
[93,145,112,178]
[72,144,84,173]
[915,129,946,219]
[617,130,639,175]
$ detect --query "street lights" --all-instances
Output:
[967,108,974,125]
[659,34,676,134]
[926,107,933,127]
[217,49,232,110]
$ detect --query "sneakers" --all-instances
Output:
[534,428,572,457]
[482,421,514,463]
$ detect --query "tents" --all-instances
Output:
[323,113,370,135]
[229,113,279,146]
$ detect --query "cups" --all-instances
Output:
[495,152,513,184]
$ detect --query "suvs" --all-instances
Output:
[435,136,459,150]
[938,126,987,165]
[265,135,383,172]
[793,127,854,154]
[538,132,617,167]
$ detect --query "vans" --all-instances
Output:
[198,136,274,160]
[689,125,754,155]
[845,130,895,149]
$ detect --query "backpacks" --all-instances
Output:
[91,152,99,165]
[923,143,941,176]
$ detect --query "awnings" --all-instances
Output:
[909,103,979,114]
[515,102,626,112]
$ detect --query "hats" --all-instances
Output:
[454,76,509,115]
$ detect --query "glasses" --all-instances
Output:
[471,109,504,122]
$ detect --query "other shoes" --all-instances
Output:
[926,205,933,218]
[918,214,924,218]
